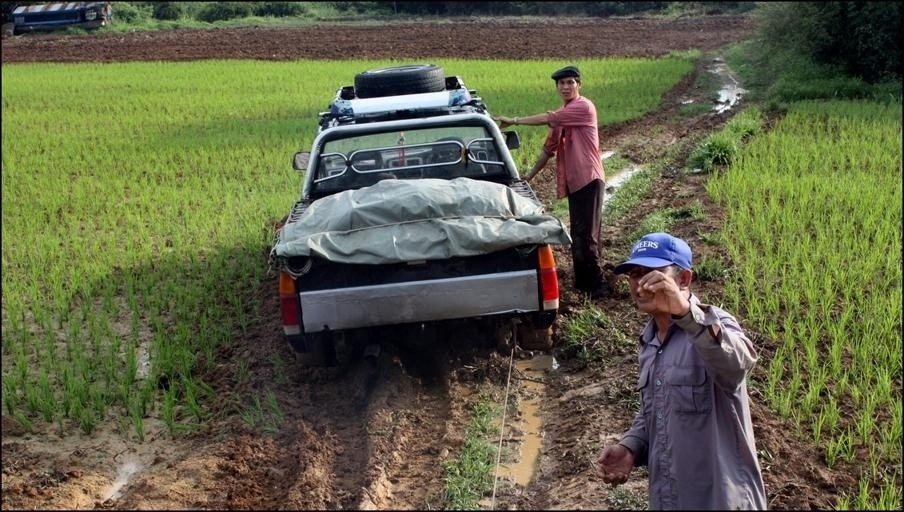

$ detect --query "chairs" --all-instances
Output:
[335,150,398,190]
[423,137,486,177]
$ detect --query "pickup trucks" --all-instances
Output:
[267,113,561,367]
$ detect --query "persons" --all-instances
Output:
[490,65,608,297]
[594,231,768,510]
[102,2,112,23]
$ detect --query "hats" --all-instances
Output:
[614,233,692,275]
[551,66,580,80]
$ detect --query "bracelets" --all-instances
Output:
[513,116,519,126]
